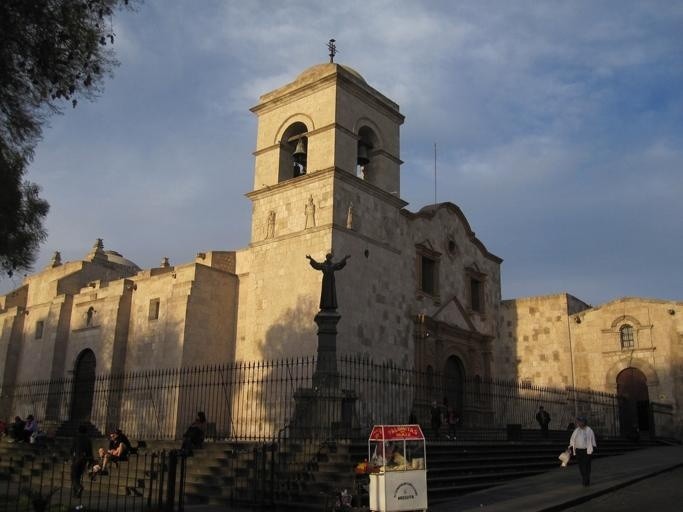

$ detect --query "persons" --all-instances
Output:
[303,249,352,311]
[6,415,26,444]
[534,406,552,434]
[428,400,443,441]
[21,414,37,443]
[380,439,405,467]
[178,410,207,452]
[565,413,596,490]
[0,414,8,439]
[444,404,458,441]
[69,428,131,499]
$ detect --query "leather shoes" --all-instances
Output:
[584,479,589,486]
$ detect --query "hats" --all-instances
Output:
[576,416,588,422]
